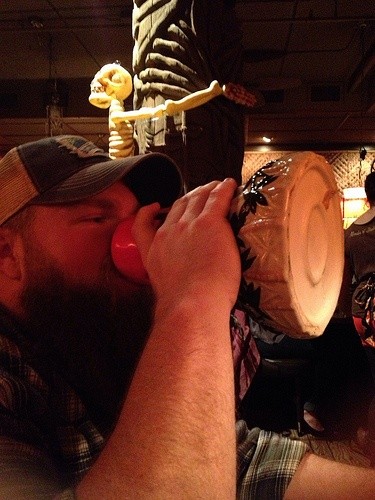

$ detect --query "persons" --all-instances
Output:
[343,169,375,367]
[0,135,375,499]
[89,61,221,160]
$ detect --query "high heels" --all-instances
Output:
[297,417,331,439]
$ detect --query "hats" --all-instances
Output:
[0,135,184,226]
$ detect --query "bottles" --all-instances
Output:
[112,150,345,339]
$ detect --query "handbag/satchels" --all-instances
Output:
[350,274,374,349]
